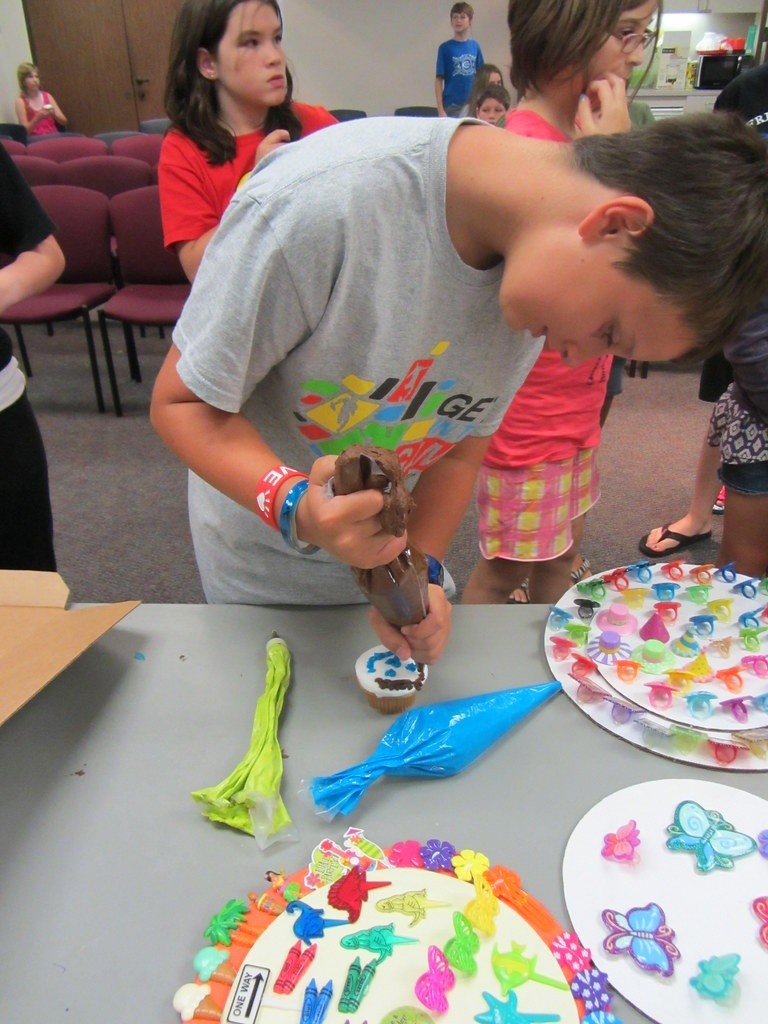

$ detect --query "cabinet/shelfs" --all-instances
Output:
[655,0,764,14]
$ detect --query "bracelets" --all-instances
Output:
[280,479,321,555]
[255,466,309,532]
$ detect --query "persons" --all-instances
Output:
[435,0,768,606]
[14,63,67,137]
[157,1,341,285]
[149,115,768,605]
[0,143,66,573]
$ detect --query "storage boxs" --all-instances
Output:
[652,31,692,90]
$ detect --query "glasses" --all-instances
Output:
[606,29,657,53]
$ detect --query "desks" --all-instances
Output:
[0,603,768,1024]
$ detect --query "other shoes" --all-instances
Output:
[712,504,724,515]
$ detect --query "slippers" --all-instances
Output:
[506,578,530,604]
[570,558,590,583]
[638,522,712,559]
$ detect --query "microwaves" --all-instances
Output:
[694,54,754,91]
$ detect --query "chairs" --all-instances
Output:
[687,96,715,118]
[394,106,440,118]
[0,119,191,418]
[328,109,366,123]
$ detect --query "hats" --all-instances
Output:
[713,63,768,140]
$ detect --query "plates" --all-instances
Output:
[543,562,767,773]
[562,780,765,1021]
